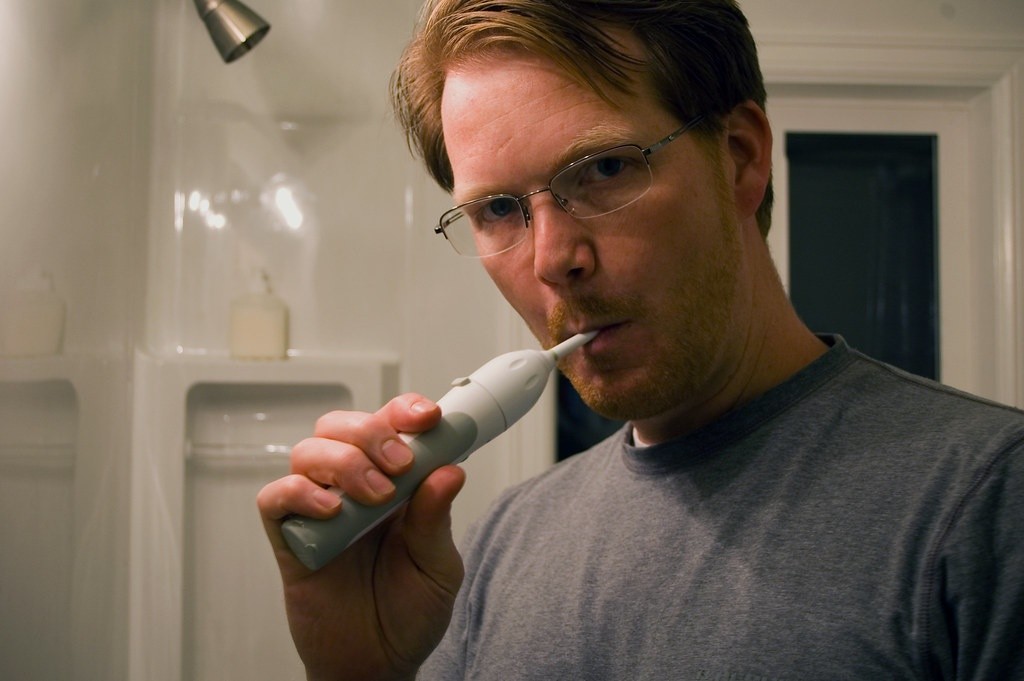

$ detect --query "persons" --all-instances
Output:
[256,0,1024,680]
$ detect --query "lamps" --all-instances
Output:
[193,0,270,63]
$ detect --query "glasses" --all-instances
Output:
[434,92,746,258]
